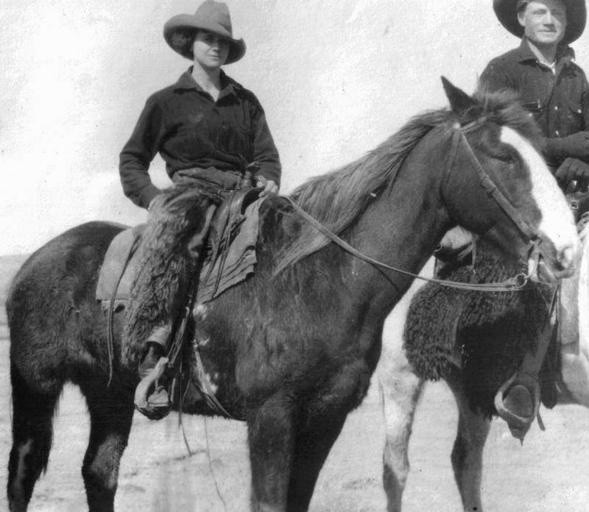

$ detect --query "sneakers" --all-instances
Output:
[504,383,538,440]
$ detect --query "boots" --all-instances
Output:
[141,345,169,407]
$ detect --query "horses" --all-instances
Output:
[370,211,589,512]
[5,74,584,512]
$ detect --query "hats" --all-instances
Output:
[164,0,246,65]
[493,0,586,44]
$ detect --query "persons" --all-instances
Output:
[113,1,285,421]
[476,0,588,433]
